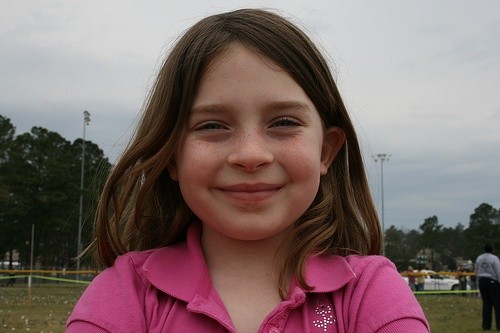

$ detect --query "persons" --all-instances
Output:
[474,244,500,330]
[459,266,478,296]
[408,265,425,291]
[66,7,432,333]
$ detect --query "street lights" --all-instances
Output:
[74,111,93,281]
[371,150,392,256]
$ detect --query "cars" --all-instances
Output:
[400,270,463,296]
